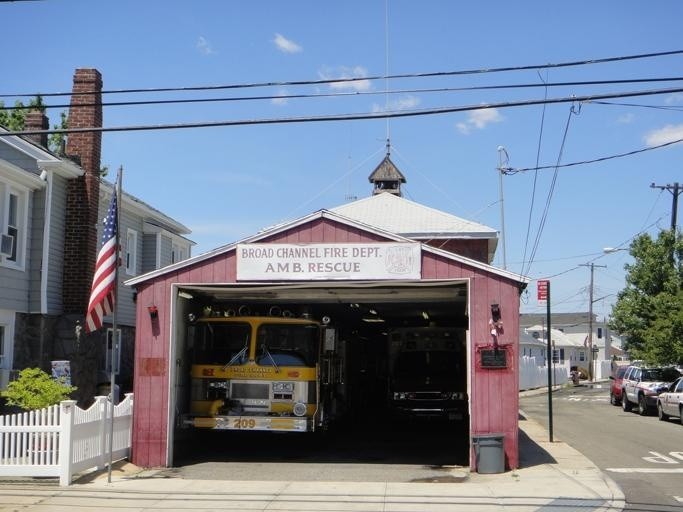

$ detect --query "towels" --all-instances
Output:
[570,371,581,387]
[98,381,119,406]
[472,435,505,474]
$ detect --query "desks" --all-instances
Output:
[601,245,627,257]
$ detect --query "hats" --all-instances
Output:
[631,360,649,368]
[609,366,629,406]
[566,370,582,380]
[656,377,683,425]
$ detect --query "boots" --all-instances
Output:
[621,366,683,416]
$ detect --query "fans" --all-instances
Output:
[480,349,508,369]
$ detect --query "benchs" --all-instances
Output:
[85,182,122,337]
